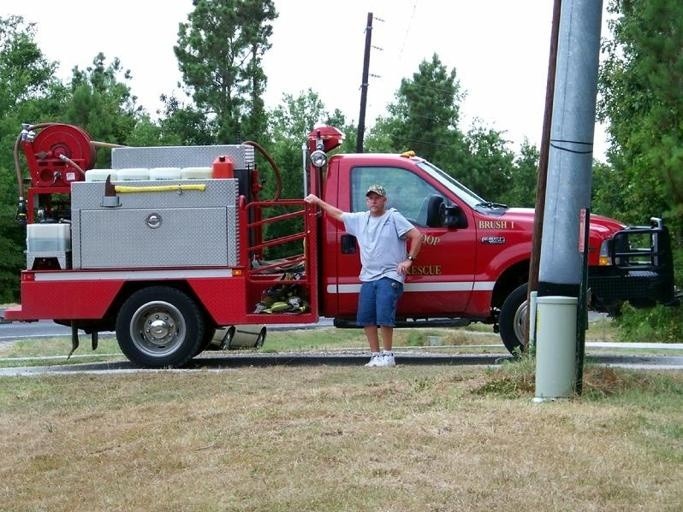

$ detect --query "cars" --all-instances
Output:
[210,325,267,350]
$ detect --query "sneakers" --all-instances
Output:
[364,352,396,367]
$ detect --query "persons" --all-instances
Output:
[303,184,422,366]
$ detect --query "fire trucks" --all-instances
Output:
[3,121,683,367]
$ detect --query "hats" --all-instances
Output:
[366,185,387,197]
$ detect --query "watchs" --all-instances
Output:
[408,255,415,261]
